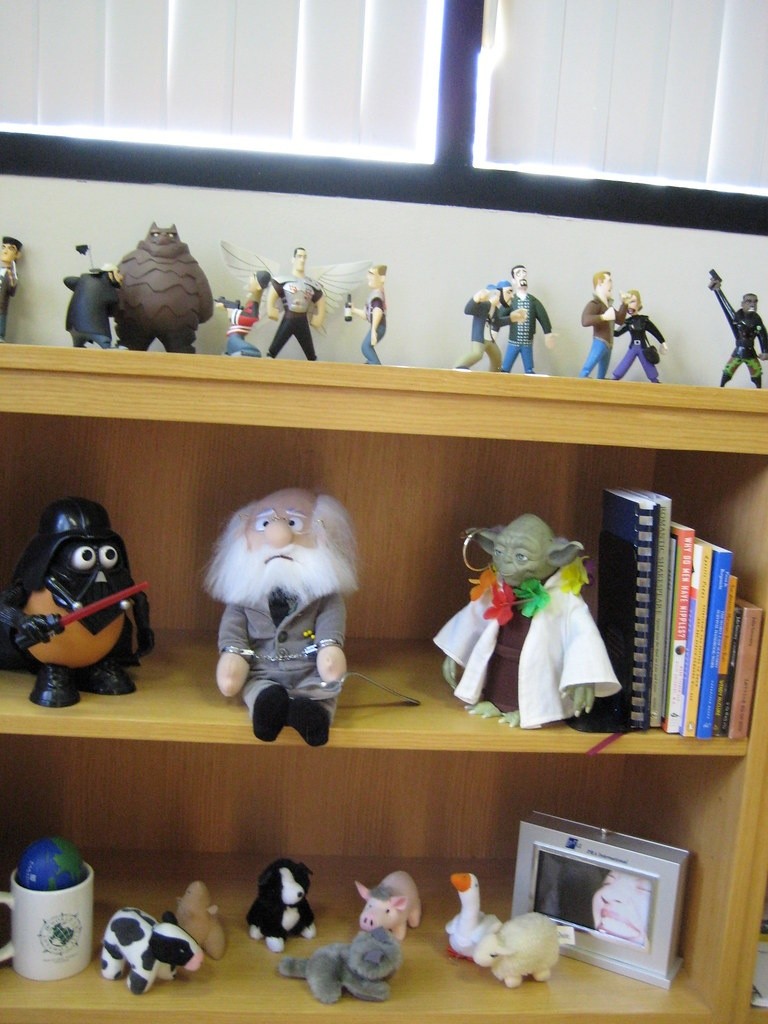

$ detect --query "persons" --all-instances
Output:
[346,263,388,366]
[492,266,558,376]
[213,268,271,357]
[589,869,654,944]
[216,236,368,363]
[454,281,530,371]
[579,272,630,379]
[708,271,768,390]
[63,264,123,349]
[609,289,669,382]
[0,235,22,344]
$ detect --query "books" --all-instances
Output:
[600,487,764,743]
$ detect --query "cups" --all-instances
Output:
[0,860,94,982]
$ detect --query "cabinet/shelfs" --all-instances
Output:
[1,342,768,1024]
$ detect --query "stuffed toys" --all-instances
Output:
[279,925,403,1003]
[474,911,561,989]
[355,870,422,941]
[444,873,504,964]
[100,907,205,995]
[245,858,316,952]
[177,881,226,959]
[206,484,361,748]
